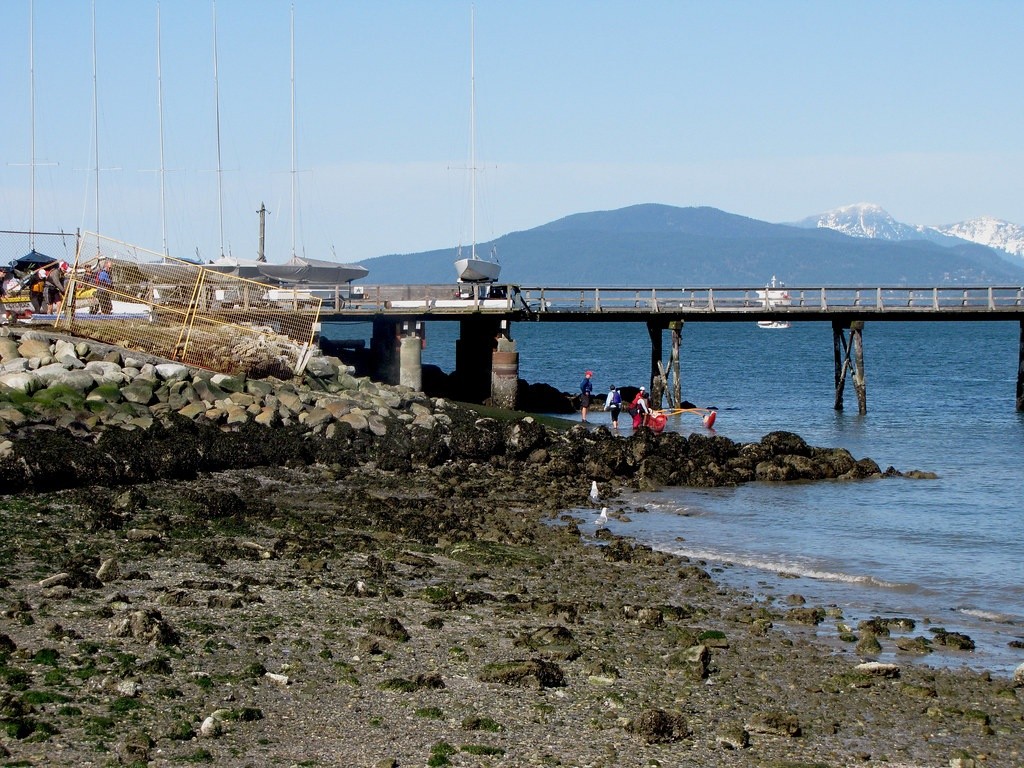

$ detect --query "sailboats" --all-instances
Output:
[454,2,504,280]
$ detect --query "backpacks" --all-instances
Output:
[613,391,621,404]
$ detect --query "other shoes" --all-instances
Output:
[581,419,590,424]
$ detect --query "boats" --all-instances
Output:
[755,276,792,329]
[0,286,99,319]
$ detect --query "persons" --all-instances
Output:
[580,371,593,424]
[0,260,113,315]
[604,384,622,429]
[628,386,650,430]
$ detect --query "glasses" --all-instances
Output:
[106,265,112,270]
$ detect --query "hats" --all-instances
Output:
[586,371,593,375]
[38,269,46,277]
[61,262,69,270]
[609,385,615,390]
[640,386,646,390]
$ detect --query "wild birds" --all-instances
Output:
[594,506,609,529]
[590,480,599,500]
